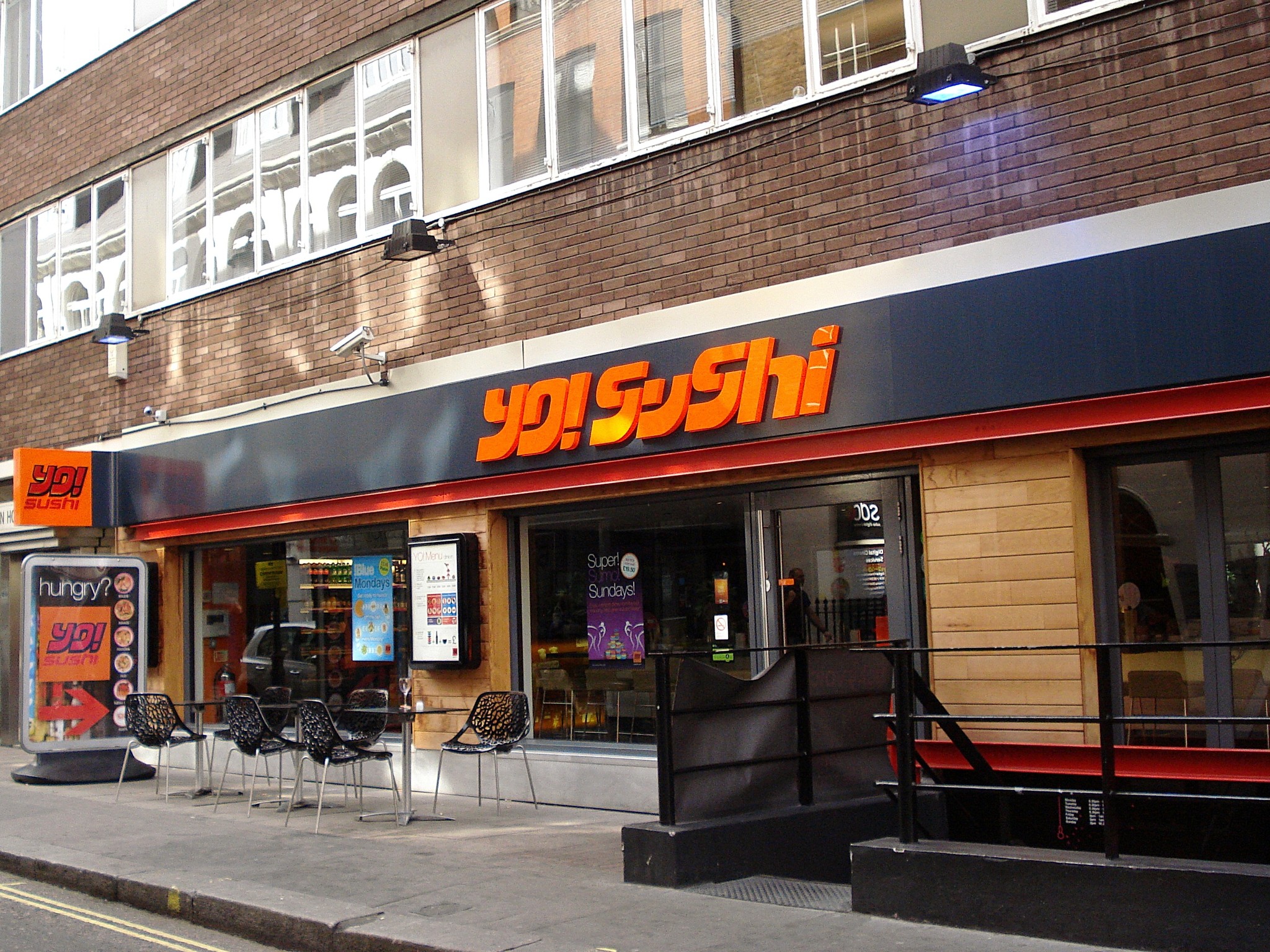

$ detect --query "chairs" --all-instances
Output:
[114,685,538,836]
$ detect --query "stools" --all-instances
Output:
[539,667,657,746]
[1126,668,1206,748]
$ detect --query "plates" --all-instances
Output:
[427,595,457,615]
[114,573,135,594]
[113,599,134,621]
[113,626,135,647]
[437,618,441,625]
[113,705,133,727]
[113,679,134,701]
[355,601,388,656]
[114,653,133,673]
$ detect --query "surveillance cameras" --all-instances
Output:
[143,406,153,415]
[330,326,374,358]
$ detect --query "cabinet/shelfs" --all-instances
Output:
[299,556,410,684]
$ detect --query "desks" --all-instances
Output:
[251,702,345,818]
[159,699,245,801]
[344,705,471,827]
[544,650,589,663]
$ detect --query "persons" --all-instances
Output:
[549,612,574,642]
[786,568,833,645]
[830,578,872,641]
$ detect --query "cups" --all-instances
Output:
[442,640,447,644]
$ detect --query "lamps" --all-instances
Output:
[89,312,150,346]
[380,218,456,263]
[900,41,1002,108]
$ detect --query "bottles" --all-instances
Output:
[436,631,438,644]
[399,678,413,709]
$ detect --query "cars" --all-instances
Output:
[240,621,327,726]
